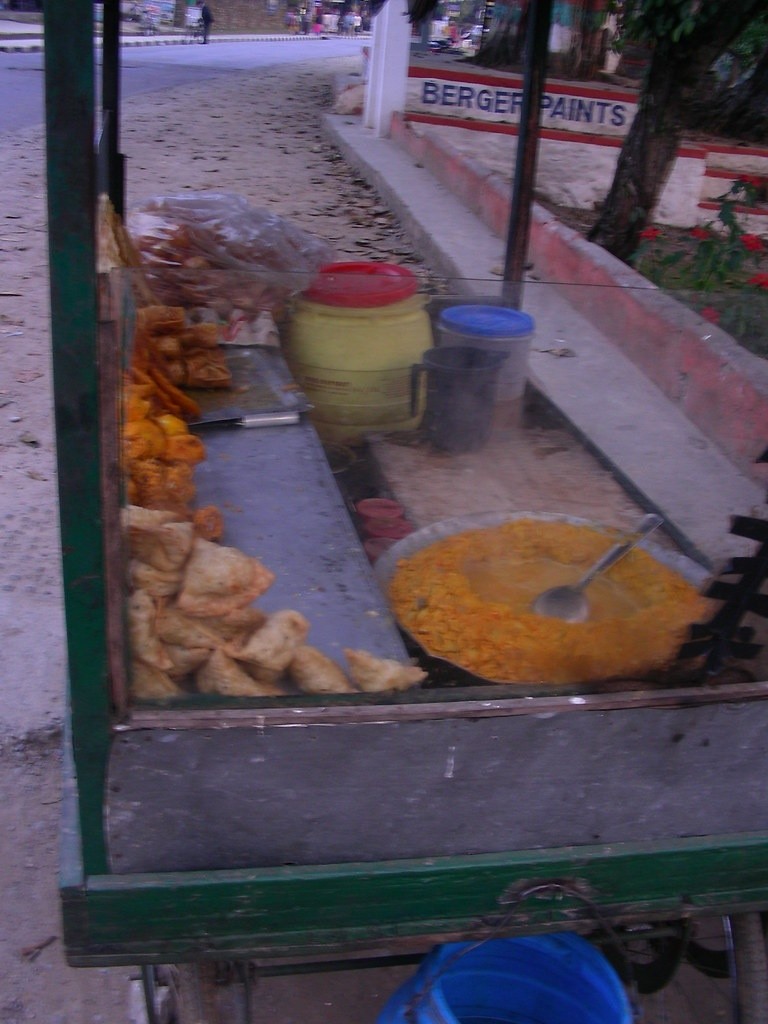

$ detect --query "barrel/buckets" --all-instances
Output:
[287,262,433,446]
[375,932,634,1024]
[435,305,535,431]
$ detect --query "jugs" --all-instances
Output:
[410,347,510,452]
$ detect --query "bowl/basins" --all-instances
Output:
[376,510,712,685]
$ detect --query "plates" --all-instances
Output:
[320,440,356,473]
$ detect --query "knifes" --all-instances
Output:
[185,412,301,430]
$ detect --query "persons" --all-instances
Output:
[196,0,214,44]
[283,6,459,41]
